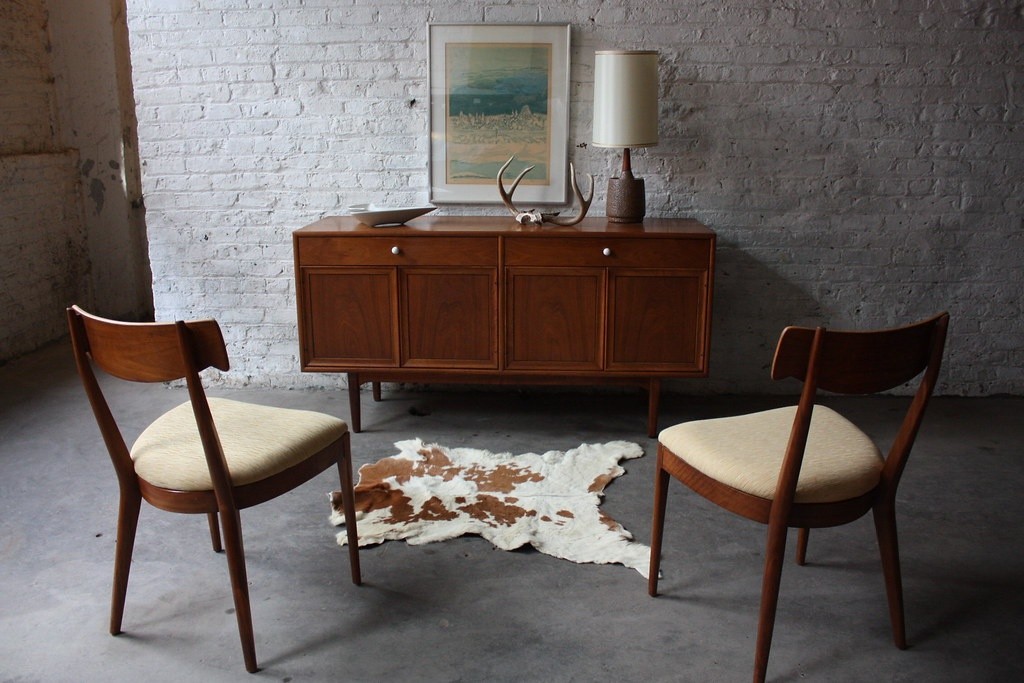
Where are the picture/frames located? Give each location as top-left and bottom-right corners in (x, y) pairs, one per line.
(427, 23), (571, 205)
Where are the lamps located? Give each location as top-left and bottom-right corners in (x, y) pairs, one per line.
(594, 47), (661, 223)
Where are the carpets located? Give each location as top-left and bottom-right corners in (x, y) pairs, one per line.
(328, 436), (663, 580)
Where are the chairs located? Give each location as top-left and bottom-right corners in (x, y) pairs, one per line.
(66, 304), (362, 674)
(647, 311), (951, 683)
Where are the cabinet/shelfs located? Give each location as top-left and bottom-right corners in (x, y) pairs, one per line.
(501, 234), (712, 376)
(298, 234), (499, 369)
